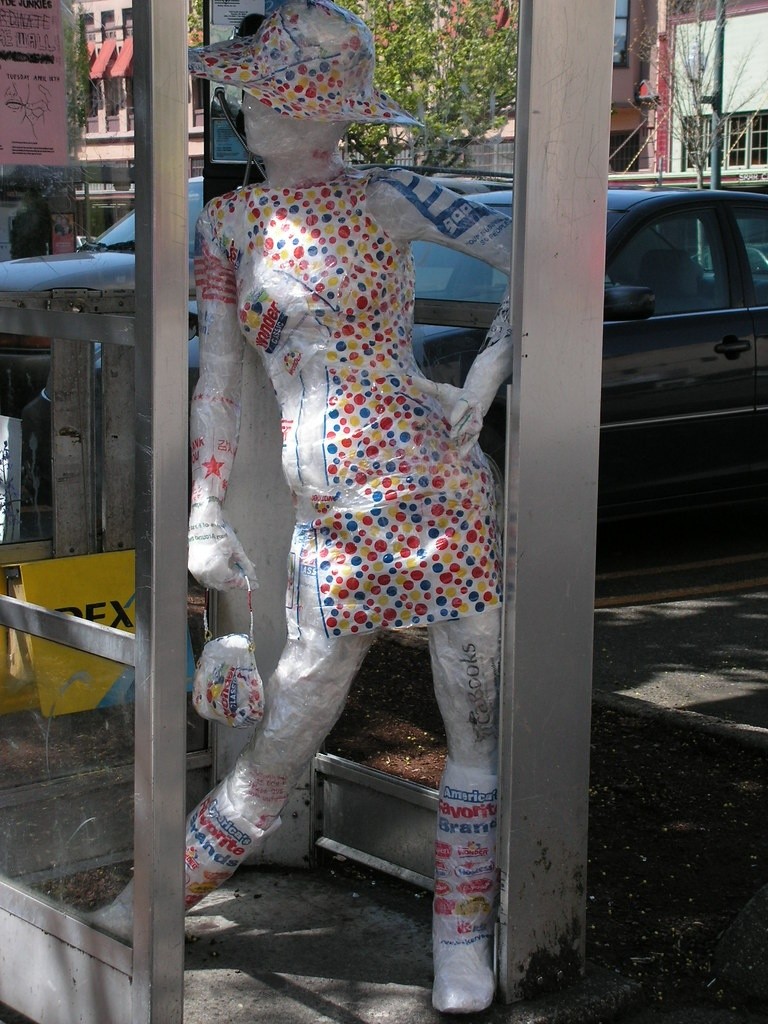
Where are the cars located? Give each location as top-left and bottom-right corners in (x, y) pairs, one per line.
(0, 173), (768, 551)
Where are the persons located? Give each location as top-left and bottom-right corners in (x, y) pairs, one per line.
(85, 3), (517, 1011)
